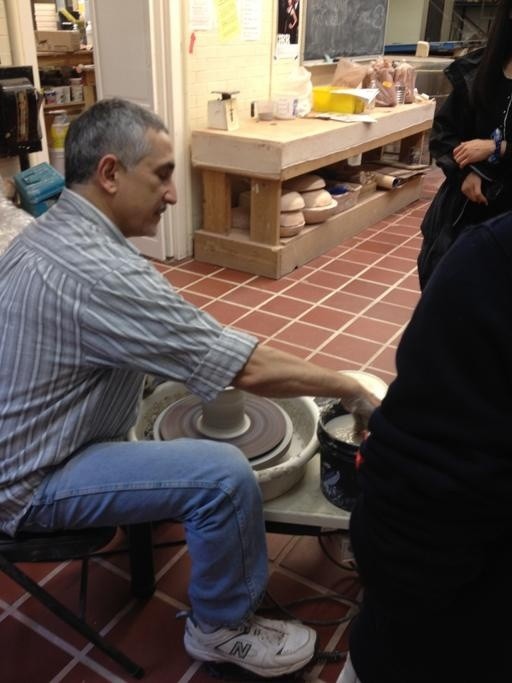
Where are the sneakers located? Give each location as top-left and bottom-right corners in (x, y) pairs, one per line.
(183, 612), (320, 678)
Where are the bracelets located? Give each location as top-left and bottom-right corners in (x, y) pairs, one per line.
(489, 126), (506, 165)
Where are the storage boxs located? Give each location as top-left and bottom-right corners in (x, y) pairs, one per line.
(36, 31), (81, 52)
(313, 86), (365, 115)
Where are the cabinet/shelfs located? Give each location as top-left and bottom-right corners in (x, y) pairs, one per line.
(37, 50), (97, 152)
(191, 101), (436, 279)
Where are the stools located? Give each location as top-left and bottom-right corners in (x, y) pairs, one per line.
(0, 524), (156, 680)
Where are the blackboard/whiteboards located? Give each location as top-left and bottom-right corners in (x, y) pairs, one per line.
(297, 0), (390, 67)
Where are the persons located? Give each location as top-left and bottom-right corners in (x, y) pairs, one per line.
(347, 211), (511, 681)
(417, 1), (512, 290)
(0, 100), (385, 679)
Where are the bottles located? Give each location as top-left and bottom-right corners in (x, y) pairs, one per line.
(86, 22), (93, 46)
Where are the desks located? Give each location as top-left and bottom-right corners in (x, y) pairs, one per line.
(124, 452), (353, 607)
